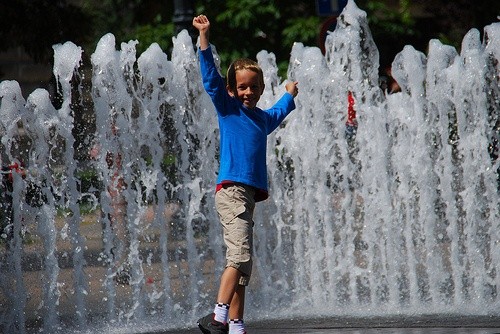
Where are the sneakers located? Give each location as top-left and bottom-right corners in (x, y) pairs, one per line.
(198, 313), (229, 334)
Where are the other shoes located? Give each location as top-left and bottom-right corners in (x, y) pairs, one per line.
(113, 266), (131, 285)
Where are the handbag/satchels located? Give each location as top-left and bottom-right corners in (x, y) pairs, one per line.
(22, 178), (57, 207)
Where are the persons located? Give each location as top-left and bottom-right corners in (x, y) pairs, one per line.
(193, 14), (299, 334)
(346, 66), (403, 163)
(0, 152), (55, 250)
(90, 113), (152, 286)
